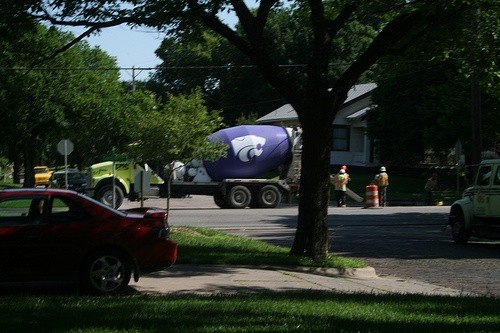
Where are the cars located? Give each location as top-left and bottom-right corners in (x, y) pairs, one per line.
(49, 168), (88, 195)
(0, 187), (178, 293)
(34, 166), (54, 188)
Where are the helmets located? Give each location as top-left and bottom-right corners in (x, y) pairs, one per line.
(380, 166), (386, 171)
(341, 165), (347, 170)
(375, 175), (379, 179)
(339, 169), (345, 173)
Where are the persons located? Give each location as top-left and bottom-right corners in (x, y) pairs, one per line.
(334, 165), (351, 207)
(376, 166), (389, 206)
(424, 172), (438, 205)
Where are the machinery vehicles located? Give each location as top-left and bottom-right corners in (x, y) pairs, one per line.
(86, 125), (303, 208)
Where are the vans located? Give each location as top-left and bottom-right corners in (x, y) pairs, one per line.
(447, 158), (500, 249)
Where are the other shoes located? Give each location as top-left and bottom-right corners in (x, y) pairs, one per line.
(380, 204), (386, 207)
(337, 204), (346, 207)
